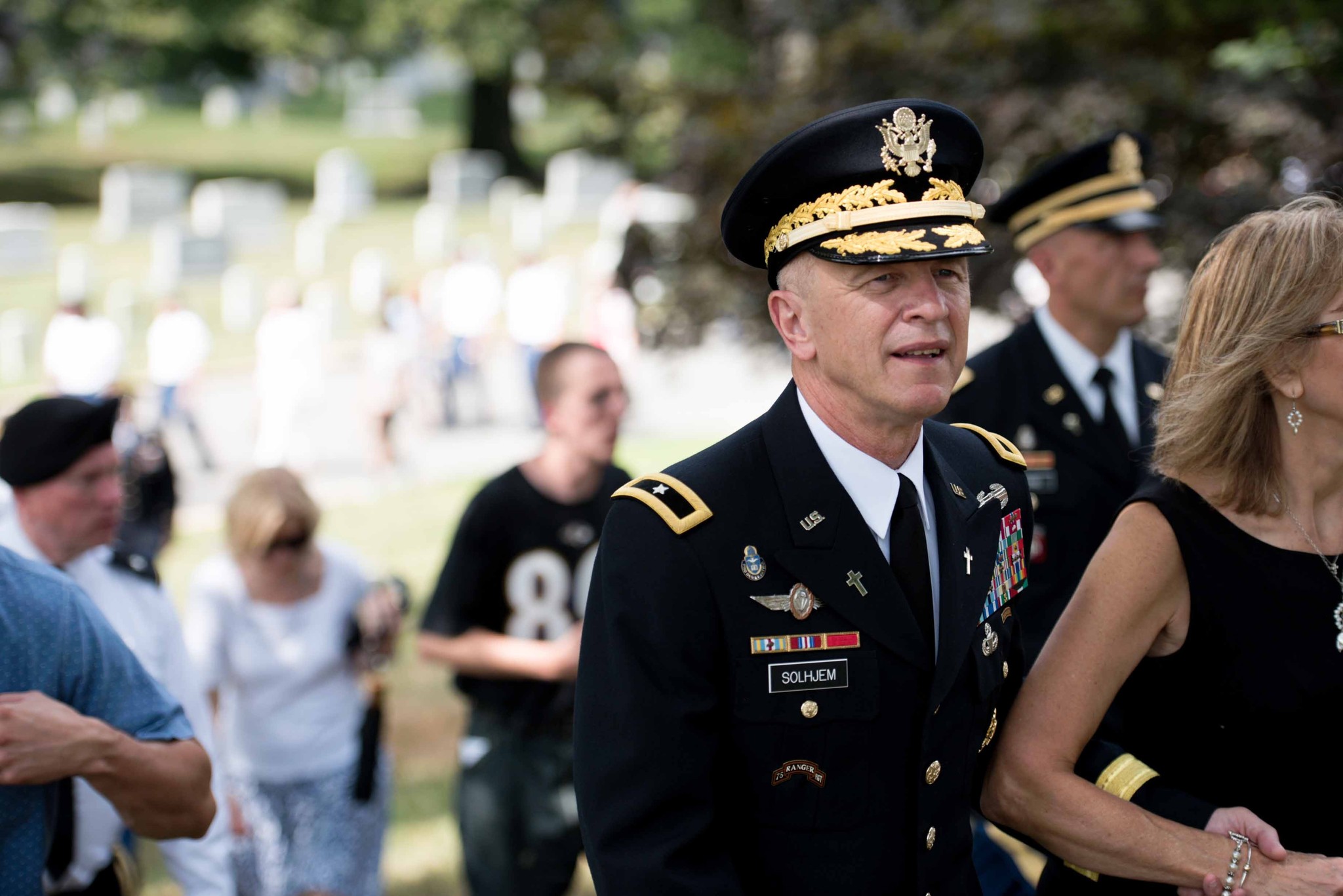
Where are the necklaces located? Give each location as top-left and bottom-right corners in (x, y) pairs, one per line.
(1273, 493), (1343, 653)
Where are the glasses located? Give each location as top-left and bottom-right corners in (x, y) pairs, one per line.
(272, 531), (314, 552)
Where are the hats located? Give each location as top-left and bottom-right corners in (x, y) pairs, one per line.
(0, 391), (125, 486)
(980, 130), (1170, 252)
(720, 95), (996, 262)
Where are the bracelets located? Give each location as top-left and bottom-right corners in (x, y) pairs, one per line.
(1221, 832), (1251, 896)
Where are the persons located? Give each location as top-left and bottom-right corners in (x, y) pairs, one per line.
(574, 99), (1287, 896)
(929, 131), (1177, 896)
(0, 283), (565, 896)
(982, 194), (1343, 896)
(416, 346), (632, 896)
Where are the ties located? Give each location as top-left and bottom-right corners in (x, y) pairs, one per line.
(885, 472), (937, 666)
(46, 776), (82, 881)
(1090, 364), (1130, 447)
